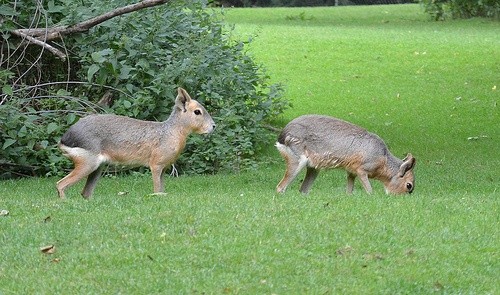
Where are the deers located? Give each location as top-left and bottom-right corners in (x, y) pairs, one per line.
(54, 86), (218, 202)
(272, 113), (417, 199)
